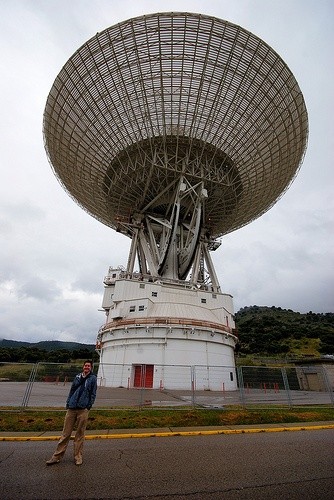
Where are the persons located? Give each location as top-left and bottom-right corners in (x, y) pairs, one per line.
(45, 360), (99, 465)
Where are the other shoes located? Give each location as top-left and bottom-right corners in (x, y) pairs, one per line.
(74, 459), (83, 464)
(46, 458), (60, 464)
